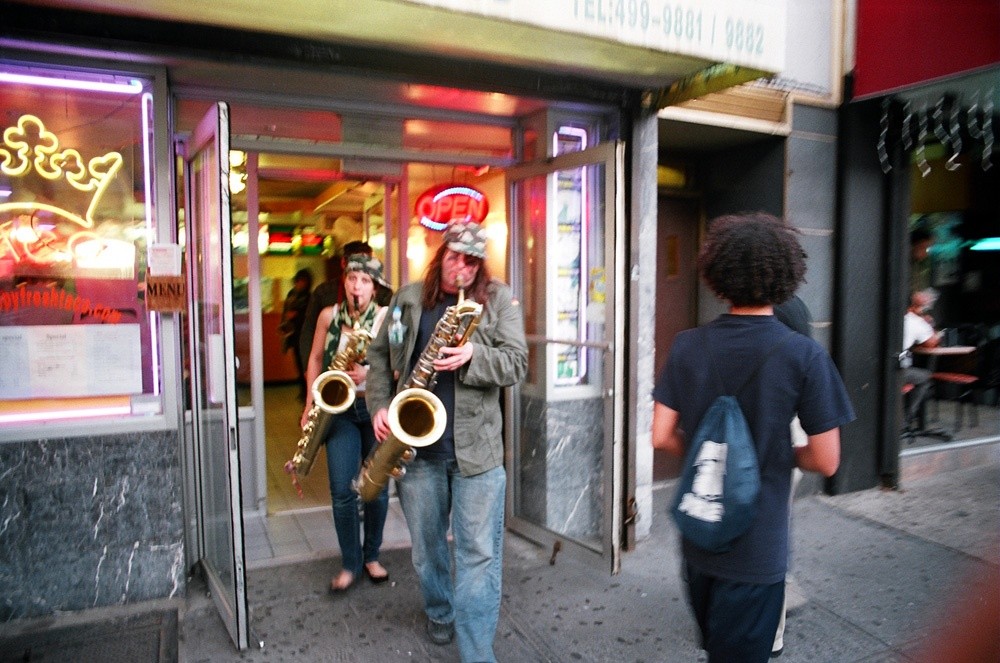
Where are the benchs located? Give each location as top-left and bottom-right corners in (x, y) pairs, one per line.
(920, 370), (978, 431)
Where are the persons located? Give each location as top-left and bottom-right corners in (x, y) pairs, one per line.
(366, 222), (528, 662)
(651, 211), (858, 663)
(280, 241), (400, 594)
(896, 229), (946, 438)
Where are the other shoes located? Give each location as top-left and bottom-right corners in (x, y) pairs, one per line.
(366, 560), (388, 584)
(426, 619), (454, 644)
(332, 569), (360, 593)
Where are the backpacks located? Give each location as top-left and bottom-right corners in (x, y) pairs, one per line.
(669, 329), (799, 550)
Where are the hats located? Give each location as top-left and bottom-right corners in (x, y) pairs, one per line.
(346, 253), (392, 289)
(443, 221), (487, 260)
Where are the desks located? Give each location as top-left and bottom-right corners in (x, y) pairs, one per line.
(910, 344), (977, 443)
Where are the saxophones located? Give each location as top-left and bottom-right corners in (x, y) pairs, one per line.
(350, 275), (484, 501)
(286, 292), (370, 476)
